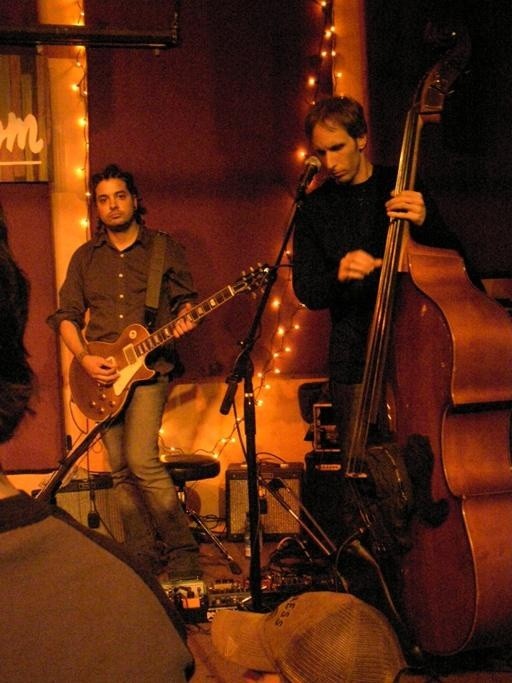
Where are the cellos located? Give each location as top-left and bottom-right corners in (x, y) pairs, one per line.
(341, 44), (512, 657)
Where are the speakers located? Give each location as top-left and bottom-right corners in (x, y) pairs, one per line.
(305, 449), (357, 543)
(225, 462), (303, 544)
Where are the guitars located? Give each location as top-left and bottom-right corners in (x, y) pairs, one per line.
(68, 264), (276, 422)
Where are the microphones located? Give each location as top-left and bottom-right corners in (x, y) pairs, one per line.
(85, 489), (103, 528)
(294, 156), (322, 200)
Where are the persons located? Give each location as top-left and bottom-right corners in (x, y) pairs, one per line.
(289, 95), (487, 448)
(44, 164), (202, 582)
(0, 206), (196, 683)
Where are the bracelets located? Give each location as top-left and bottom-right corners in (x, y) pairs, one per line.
(73, 351), (89, 362)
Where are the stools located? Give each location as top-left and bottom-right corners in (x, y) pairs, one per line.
(159, 453), (242, 574)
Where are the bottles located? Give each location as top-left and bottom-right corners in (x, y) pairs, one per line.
(244, 511), (252, 558)
